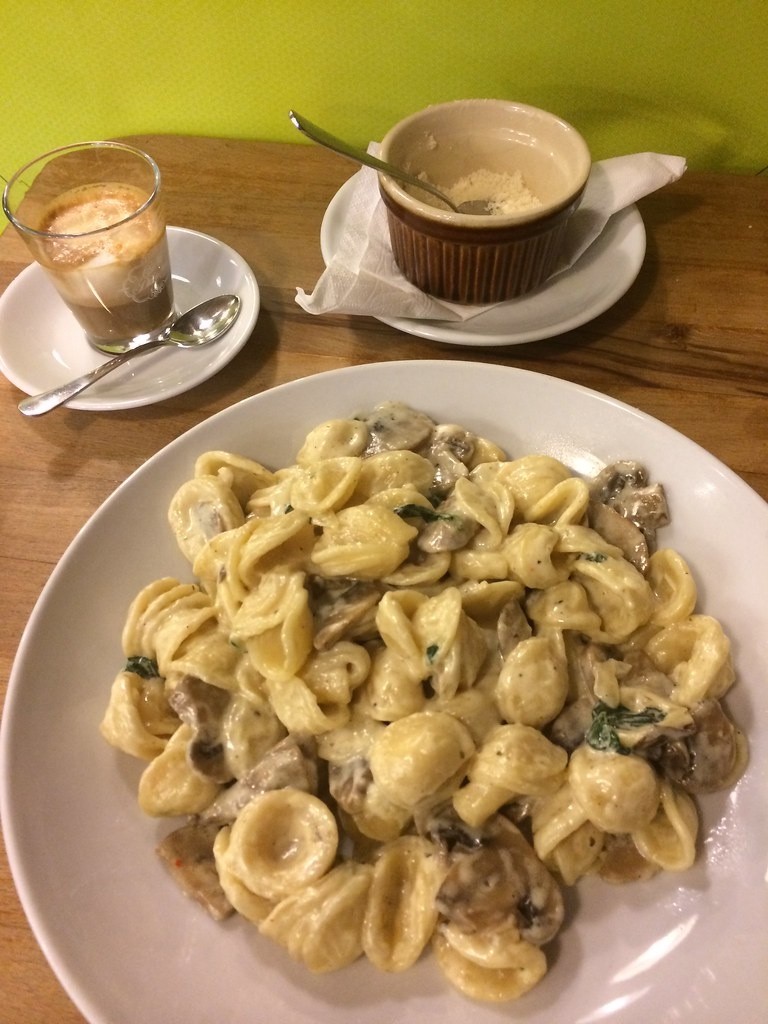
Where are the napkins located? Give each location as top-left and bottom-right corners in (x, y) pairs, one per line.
(294, 138), (689, 322)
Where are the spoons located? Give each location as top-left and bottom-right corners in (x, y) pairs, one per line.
(288, 109), (500, 216)
(18, 295), (242, 416)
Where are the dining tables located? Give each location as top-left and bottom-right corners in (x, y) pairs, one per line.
(2, 134), (768, 1024)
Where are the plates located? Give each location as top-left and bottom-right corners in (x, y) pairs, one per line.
(1, 359), (767, 1022)
(320, 165), (647, 347)
(1, 225), (260, 410)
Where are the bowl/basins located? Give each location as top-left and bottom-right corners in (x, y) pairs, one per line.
(377, 98), (591, 305)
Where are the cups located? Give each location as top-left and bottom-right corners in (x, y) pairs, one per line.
(1, 140), (180, 357)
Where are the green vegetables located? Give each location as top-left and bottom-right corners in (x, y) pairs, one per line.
(124, 502), (667, 756)
